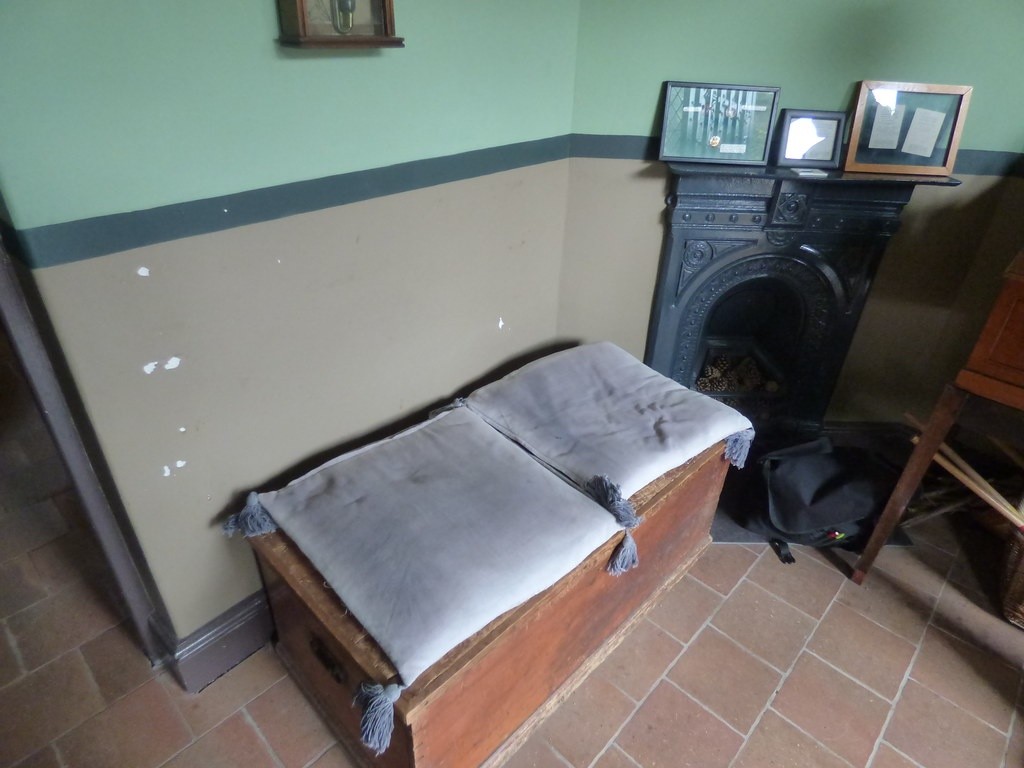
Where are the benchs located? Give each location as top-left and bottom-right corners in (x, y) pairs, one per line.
(223, 339), (755, 768)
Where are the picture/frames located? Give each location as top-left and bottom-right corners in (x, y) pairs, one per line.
(845, 79), (974, 176)
(776, 109), (846, 169)
(659, 81), (781, 167)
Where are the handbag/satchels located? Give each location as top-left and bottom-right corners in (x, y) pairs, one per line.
(752, 437), (909, 563)
